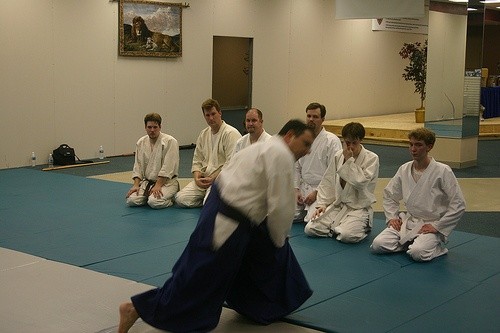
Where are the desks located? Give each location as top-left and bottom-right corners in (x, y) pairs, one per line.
(479, 85), (500, 119)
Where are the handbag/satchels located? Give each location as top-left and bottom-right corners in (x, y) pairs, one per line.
(52, 144), (93, 166)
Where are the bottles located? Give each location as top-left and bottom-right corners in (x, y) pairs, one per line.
(99, 146), (104, 159)
(48, 153), (53, 168)
(32, 152), (36, 167)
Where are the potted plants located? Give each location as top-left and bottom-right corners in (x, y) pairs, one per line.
(398, 39), (427, 124)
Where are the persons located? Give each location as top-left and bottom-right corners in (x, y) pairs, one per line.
(369, 128), (466, 262)
(174, 99), (242, 209)
(231, 109), (272, 159)
(118, 120), (313, 333)
(290, 102), (379, 244)
(125, 112), (181, 208)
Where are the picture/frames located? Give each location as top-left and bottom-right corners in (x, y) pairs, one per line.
(117, 0), (184, 57)
